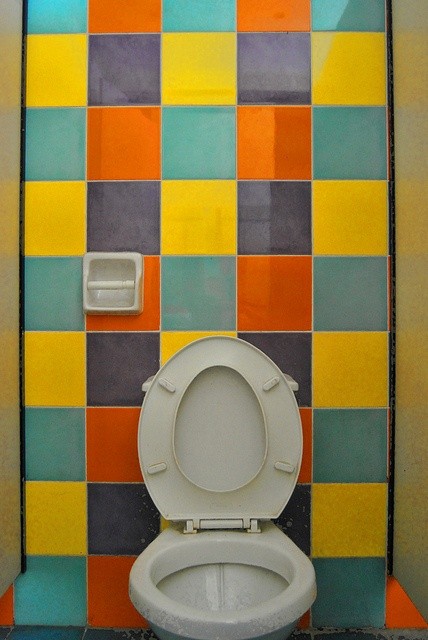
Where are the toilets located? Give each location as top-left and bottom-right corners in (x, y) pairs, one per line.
(127, 335), (319, 640)
